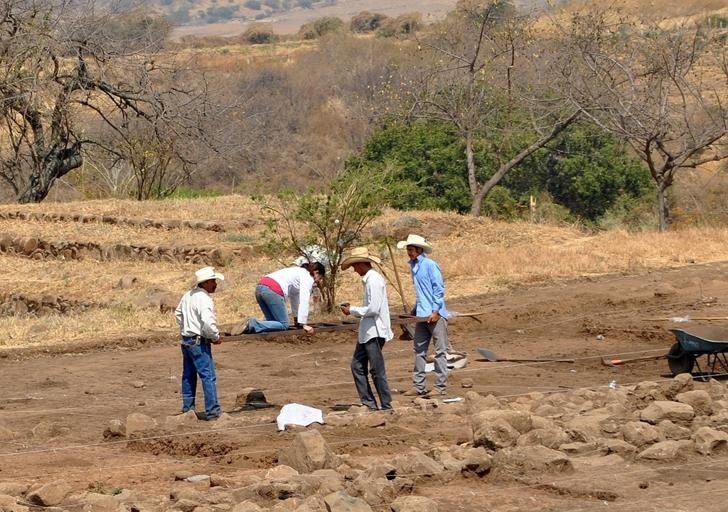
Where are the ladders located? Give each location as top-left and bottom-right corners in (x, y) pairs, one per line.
(222, 314), (430, 342)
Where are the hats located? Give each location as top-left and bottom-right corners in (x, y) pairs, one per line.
(191, 267), (225, 289)
(397, 233), (432, 255)
(341, 247), (381, 270)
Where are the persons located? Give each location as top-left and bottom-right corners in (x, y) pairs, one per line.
(229, 261), (327, 336)
(173, 265), (226, 423)
(338, 245), (397, 411)
(395, 234), (450, 398)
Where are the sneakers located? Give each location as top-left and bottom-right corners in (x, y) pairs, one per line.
(404, 387), (447, 396)
(230, 315), (255, 336)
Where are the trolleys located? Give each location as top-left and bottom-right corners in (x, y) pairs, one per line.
(663, 327), (728, 381)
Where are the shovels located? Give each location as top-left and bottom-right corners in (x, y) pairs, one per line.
(385, 236), (415, 341)
(477, 348), (575, 363)
